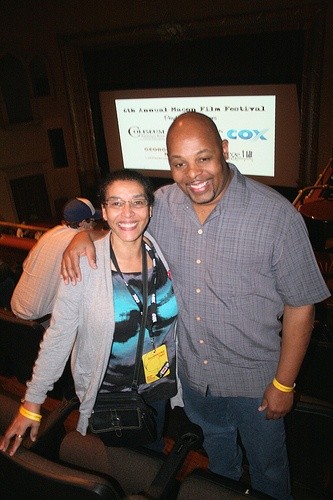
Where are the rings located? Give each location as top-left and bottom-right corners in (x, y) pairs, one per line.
(16, 434), (23, 438)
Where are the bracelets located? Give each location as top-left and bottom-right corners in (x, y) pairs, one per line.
(272, 376), (295, 393)
(19, 404), (42, 422)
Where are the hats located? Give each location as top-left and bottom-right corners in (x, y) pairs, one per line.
(64, 197), (103, 221)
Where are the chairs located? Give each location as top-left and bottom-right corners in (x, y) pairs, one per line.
(0, 233), (333, 500)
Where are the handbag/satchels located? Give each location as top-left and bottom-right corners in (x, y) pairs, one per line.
(89, 391), (164, 453)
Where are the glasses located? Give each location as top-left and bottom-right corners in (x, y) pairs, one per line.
(103, 200), (154, 209)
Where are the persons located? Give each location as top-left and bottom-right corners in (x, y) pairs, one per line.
(61, 111), (331, 500)
(11, 197), (101, 400)
(0, 169), (184, 455)
